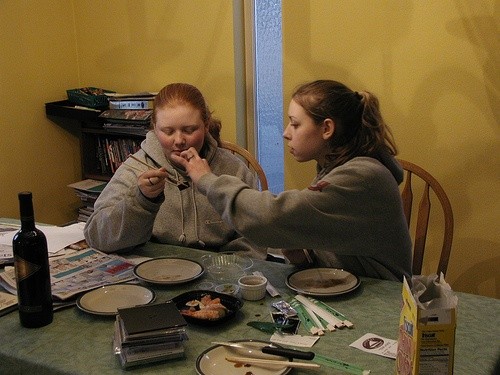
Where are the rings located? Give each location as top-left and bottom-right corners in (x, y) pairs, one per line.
(148, 178), (154, 185)
(188, 154), (194, 160)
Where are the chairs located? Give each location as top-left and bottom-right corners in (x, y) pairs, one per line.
(217, 139), (454, 283)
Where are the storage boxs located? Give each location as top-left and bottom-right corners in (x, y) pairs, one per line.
(46, 86), (116, 123)
(396, 272), (458, 375)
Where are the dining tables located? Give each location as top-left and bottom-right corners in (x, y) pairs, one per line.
(0, 218), (500, 375)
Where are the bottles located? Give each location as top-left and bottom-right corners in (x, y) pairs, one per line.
(12, 191), (54, 329)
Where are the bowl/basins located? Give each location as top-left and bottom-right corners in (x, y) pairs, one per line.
(215, 284), (239, 297)
(200, 253), (255, 281)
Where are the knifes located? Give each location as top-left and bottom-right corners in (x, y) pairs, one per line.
(210, 342), (315, 360)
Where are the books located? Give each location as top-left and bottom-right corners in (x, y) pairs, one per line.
(94, 136), (142, 175)
(68, 178), (108, 222)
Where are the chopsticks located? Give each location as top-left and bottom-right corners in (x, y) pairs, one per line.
(311, 322), (353, 336)
(225, 357), (322, 368)
(123, 154), (188, 188)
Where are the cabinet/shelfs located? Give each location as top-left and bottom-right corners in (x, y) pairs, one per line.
(77, 125), (156, 208)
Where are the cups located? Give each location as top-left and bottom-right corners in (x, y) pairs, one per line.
(238, 275), (268, 300)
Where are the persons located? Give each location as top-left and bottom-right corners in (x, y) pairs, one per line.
(170, 79), (415, 284)
(83, 83), (268, 260)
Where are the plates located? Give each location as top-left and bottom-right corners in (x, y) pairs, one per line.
(75, 283), (156, 316)
(132, 256), (206, 284)
(166, 289), (245, 325)
(193, 339), (293, 375)
(285, 266), (362, 296)
